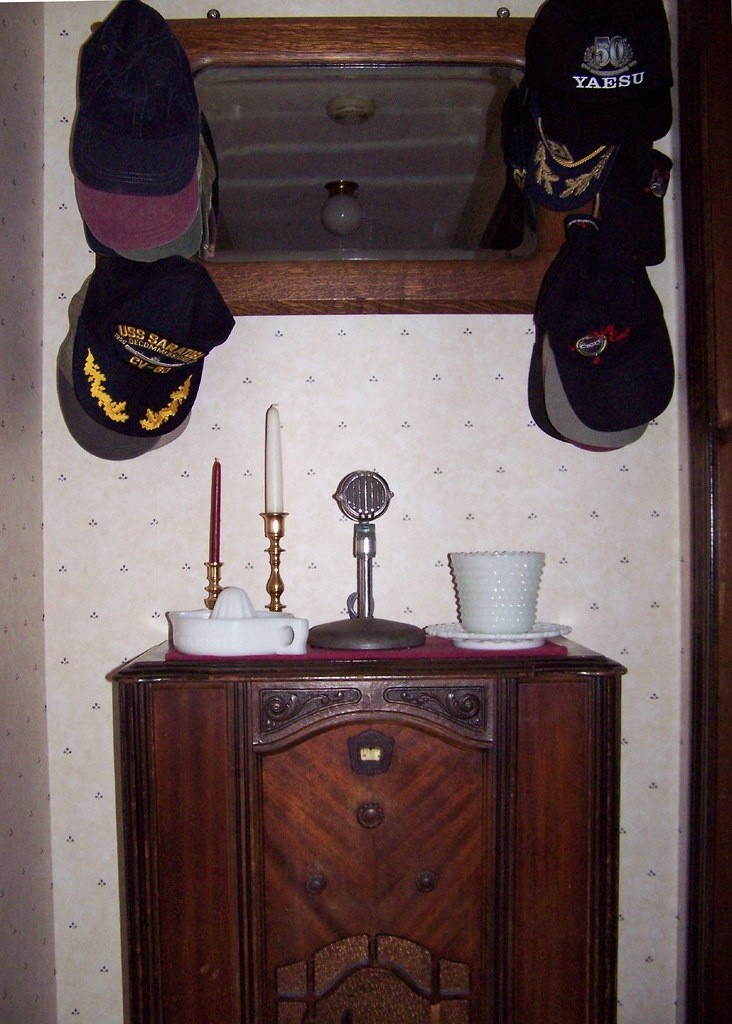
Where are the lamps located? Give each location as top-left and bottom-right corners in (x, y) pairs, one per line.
(322, 181), (363, 236)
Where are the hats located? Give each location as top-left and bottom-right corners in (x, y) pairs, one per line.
(69, 0), (202, 194)
(55, 253), (237, 461)
(476, 0), (673, 264)
(71, 114), (220, 261)
(527, 211), (678, 451)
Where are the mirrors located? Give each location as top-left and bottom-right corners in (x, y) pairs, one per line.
(163, 17), (602, 316)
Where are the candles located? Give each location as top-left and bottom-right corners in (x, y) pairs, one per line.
(209, 458), (221, 563)
(266, 404), (284, 513)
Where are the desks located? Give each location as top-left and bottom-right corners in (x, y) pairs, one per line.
(104, 636), (627, 1024)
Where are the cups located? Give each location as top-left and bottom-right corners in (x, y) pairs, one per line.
(448, 549), (547, 635)
(165, 607), (310, 658)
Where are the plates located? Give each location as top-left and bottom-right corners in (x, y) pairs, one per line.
(425, 620), (572, 650)
